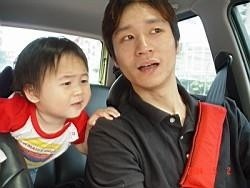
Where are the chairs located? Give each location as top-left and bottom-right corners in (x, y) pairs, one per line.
(0, 83), (111, 188)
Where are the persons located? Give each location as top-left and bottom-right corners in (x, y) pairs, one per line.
(84, 0), (250, 188)
(0, 37), (121, 186)
